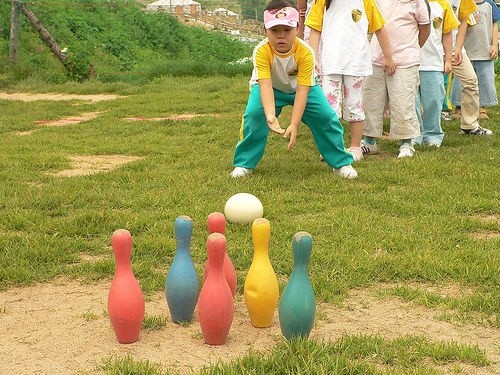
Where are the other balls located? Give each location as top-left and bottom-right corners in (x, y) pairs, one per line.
(223, 193), (263, 226)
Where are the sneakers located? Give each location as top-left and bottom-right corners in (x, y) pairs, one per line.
(346, 149), (364, 162)
(320, 154), (326, 162)
(411, 139), (423, 147)
(360, 140), (377, 155)
(478, 107), (489, 119)
(427, 142), (441, 148)
(397, 142), (415, 159)
(458, 125), (492, 136)
(230, 167), (254, 179)
(441, 112), (455, 121)
(332, 164), (359, 179)
(452, 109), (461, 118)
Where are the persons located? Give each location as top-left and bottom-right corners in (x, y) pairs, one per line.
(229, 1), (358, 180)
(297, 0), (500, 163)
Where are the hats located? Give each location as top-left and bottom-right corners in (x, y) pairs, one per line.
(263, 6), (299, 29)
